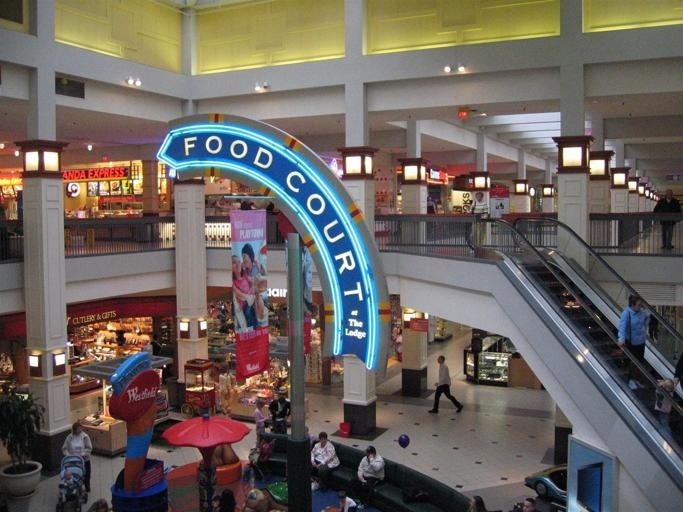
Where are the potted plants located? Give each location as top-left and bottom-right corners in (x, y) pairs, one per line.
(0, 372), (46, 498)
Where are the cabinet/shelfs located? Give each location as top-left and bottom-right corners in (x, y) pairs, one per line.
(462, 333), (545, 393)
(0, 304), (324, 458)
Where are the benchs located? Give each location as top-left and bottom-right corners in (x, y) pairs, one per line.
(253, 431), (472, 512)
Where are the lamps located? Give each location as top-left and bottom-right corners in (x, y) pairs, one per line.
(336, 145), (381, 181)
(253, 81), (269, 91)
(468, 170), (491, 190)
(13, 138), (70, 178)
(444, 62), (466, 74)
(396, 157), (430, 186)
(551, 135), (661, 201)
(540, 183), (555, 198)
(512, 178), (530, 195)
(126, 77), (143, 86)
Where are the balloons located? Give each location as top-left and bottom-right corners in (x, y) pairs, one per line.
(399, 435), (410, 449)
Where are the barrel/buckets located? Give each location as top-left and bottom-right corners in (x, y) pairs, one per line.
(340, 422), (351, 437)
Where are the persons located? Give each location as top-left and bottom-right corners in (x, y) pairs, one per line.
(513, 498), (543, 512)
(652, 188), (683, 250)
(70, 184), (80, 197)
(310, 431), (341, 491)
(469, 495), (489, 512)
(232, 242), (268, 334)
(210, 301), (235, 339)
(303, 261), (313, 317)
(60, 469), (79, 503)
(618, 294), (683, 437)
(248, 396), (291, 481)
(350, 445), (386, 509)
(470, 192), (489, 248)
(213, 489), (242, 512)
(337, 490), (358, 512)
(427, 196), (437, 234)
(428, 356), (463, 413)
(61, 422), (93, 492)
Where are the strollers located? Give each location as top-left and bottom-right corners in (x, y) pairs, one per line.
(54, 454), (91, 511)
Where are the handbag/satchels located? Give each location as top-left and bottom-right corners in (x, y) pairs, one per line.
(621, 340), (633, 361)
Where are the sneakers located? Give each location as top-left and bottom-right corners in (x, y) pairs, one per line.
(456, 405), (464, 413)
(428, 409), (439, 414)
(629, 379), (646, 391)
